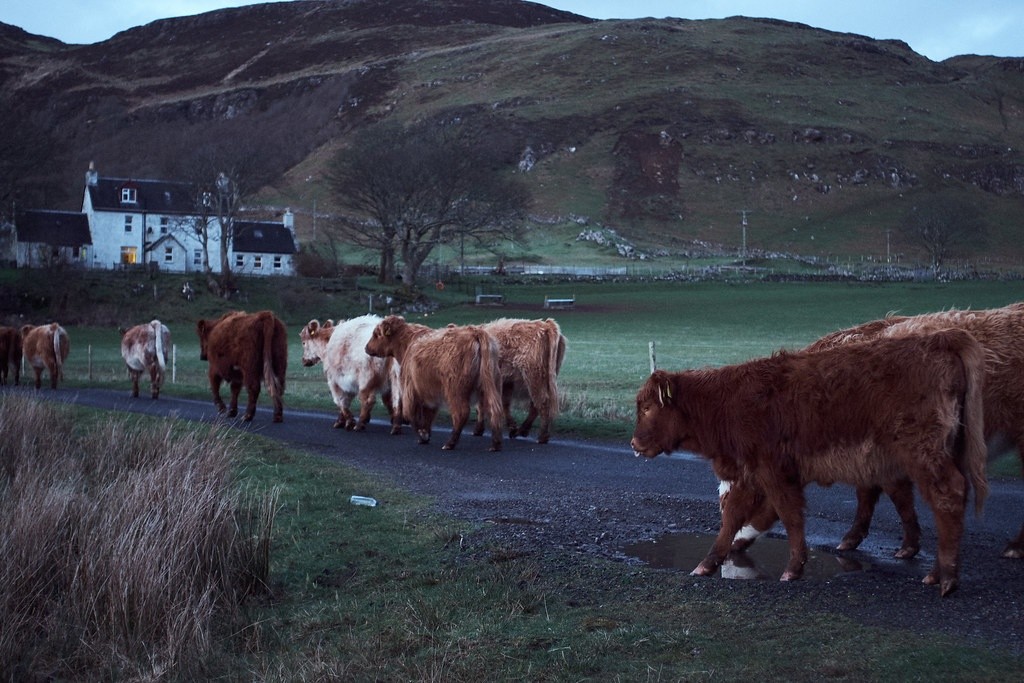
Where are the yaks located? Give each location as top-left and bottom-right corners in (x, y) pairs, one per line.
(630, 301), (1024, 597)
(0, 311), (567, 453)
